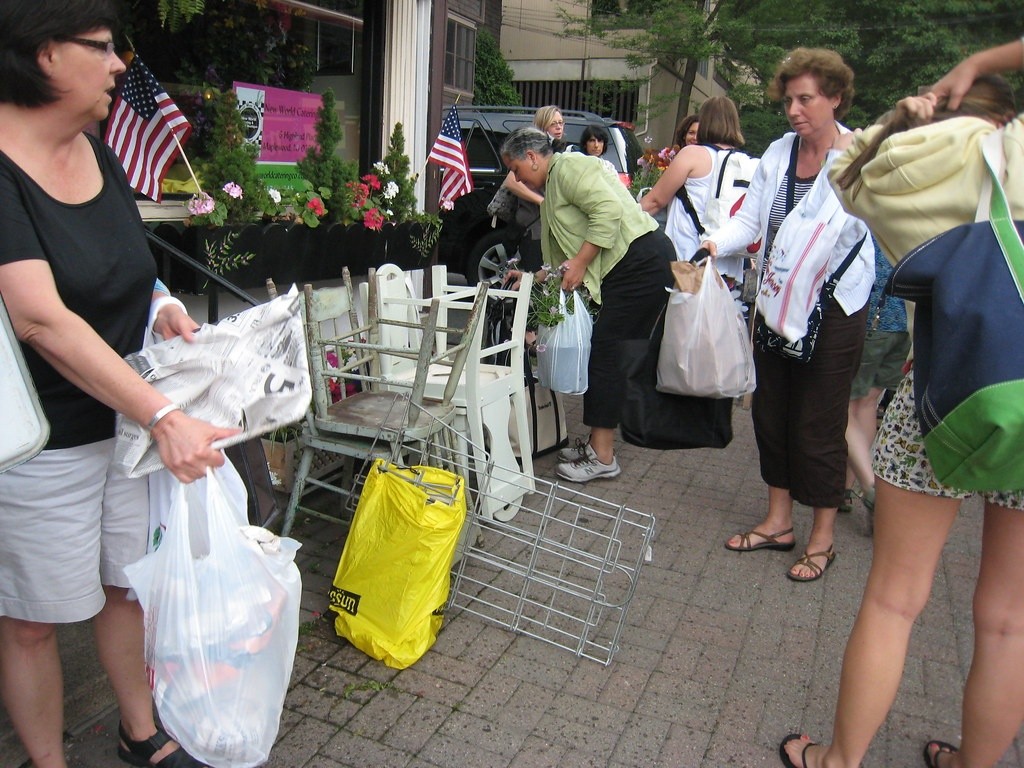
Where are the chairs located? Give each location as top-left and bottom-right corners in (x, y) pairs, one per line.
(284, 263), (532, 568)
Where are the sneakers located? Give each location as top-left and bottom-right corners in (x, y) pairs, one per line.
(556, 434), (597, 462)
(556, 445), (621, 482)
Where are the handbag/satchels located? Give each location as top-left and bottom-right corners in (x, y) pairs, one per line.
(656, 254), (758, 399)
(0, 295), (49, 476)
(888, 127), (1024, 492)
(534, 284), (593, 395)
(757, 187), (848, 342)
(122, 468), (302, 768)
(486, 180), (516, 224)
(620, 251), (734, 450)
(328, 459), (467, 670)
(754, 294), (824, 378)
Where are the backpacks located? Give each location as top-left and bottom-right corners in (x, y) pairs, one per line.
(675, 142), (762, 256)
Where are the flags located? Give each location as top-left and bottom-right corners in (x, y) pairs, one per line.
(102, 50), (193, 204)
(428, 105), (473, 211)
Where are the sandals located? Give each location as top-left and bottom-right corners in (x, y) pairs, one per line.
(780, 735), (820, 768)
(725, 528), (795, 551)
(118, 724), (202, 767)
(786, 544), (836, 582)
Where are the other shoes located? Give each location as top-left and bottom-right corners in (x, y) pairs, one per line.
(837, 489), (853, 513)
(862, 491), (875, 509)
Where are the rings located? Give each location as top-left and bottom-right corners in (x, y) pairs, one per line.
(571, 286), (575, 290)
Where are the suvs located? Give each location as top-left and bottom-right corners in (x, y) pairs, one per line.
(441, 104), (649, 314)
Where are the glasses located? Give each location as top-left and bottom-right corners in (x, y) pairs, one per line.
(550, 121), (564, 129)
(64, 35), (114, 57)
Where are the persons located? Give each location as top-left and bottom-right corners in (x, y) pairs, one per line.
(483, 102), (678, 483)
(778, 35), (1024, 768)
(701, 50), (878, 583)
(636, 96), (759, 289)
(0, 0), (239, 768)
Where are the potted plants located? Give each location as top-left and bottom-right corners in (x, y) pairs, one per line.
(261, 401), (344, 492)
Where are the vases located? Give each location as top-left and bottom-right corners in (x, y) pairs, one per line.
(187, 223), (437, 294)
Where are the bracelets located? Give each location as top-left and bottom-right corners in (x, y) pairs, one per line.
(146, 403), (182, 432)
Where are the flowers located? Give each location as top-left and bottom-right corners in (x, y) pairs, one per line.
(630, 137), (681, 203)
(182, 161), (445, 257)
(507, 258), (568, 352)
(325, 337), (366, 403)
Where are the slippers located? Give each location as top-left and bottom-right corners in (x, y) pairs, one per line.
(924, 739), (959, 767)
